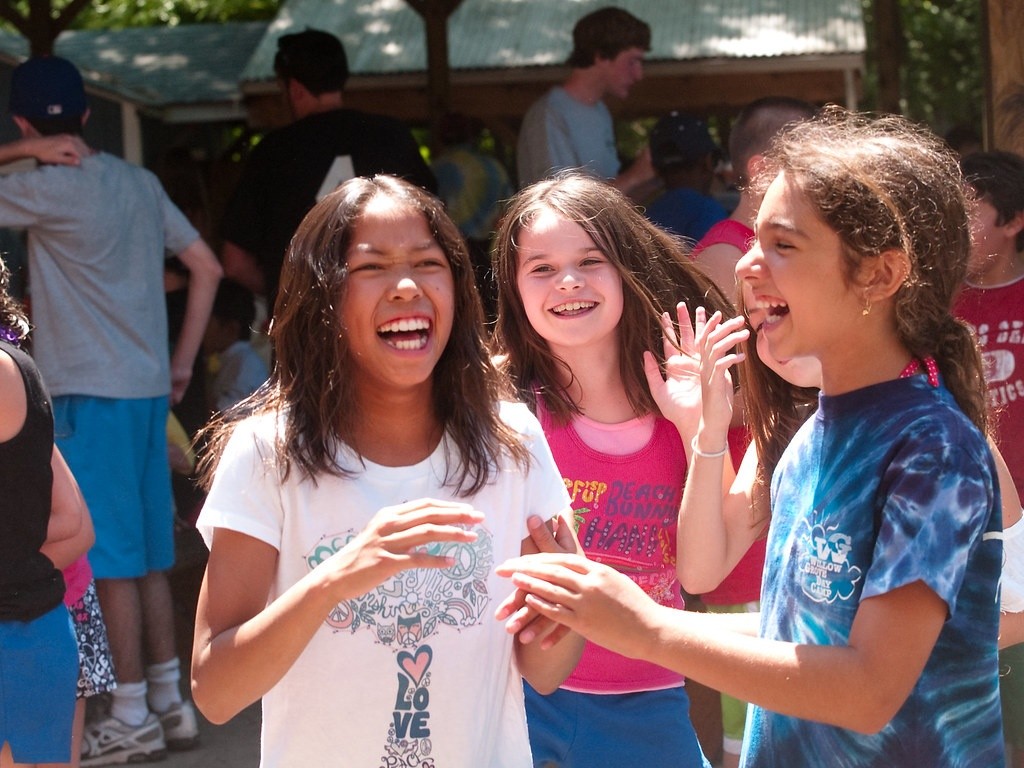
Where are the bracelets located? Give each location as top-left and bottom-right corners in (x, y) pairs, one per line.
(691, 436), (728, 458)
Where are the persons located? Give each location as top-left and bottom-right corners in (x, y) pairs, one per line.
(191, 176), (585, 768)
(492, 177), (736, 768)
(497, 128), (1008, 768)
(645, 96), (1024, 768)
(0, 29), (437, 768)
(518, 8), (651, 196)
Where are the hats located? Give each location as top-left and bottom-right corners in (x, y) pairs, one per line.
(11, 54), (87, 116)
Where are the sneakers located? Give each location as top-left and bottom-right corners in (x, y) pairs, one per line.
(150, 700), (201, 751)
(80, 709), (166, 767)
